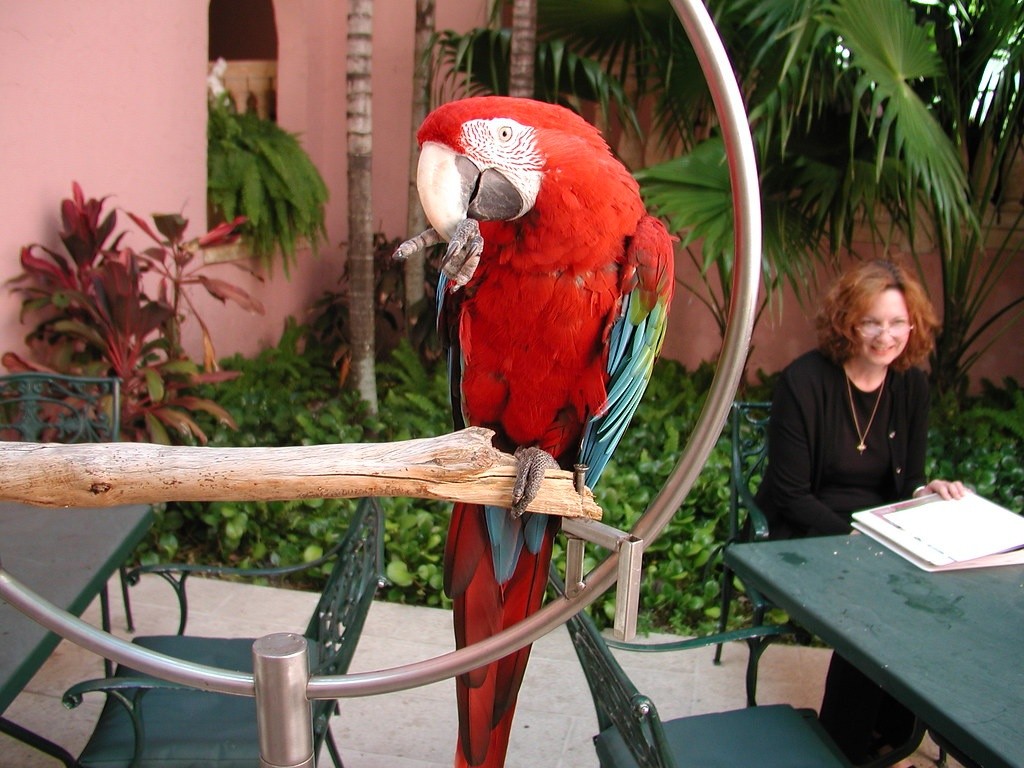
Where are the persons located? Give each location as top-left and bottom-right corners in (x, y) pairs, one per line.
(736, 255), (965, 768)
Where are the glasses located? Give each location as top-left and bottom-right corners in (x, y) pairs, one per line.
(854, 316), (914, 337)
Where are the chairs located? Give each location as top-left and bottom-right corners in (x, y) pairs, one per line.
(713, 399), (950, 764)
(0, 370), (136, 678)
(549, 561), (855, 768)
(62, 495), (384, 768)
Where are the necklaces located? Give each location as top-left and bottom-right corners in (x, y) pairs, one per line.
(843, 365), (886, 455)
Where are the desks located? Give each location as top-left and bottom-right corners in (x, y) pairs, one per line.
(721, 534), (1024, 768)
(0, 498), (156, 768)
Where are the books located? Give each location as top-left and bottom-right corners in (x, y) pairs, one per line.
(850, 489), (1024, 573)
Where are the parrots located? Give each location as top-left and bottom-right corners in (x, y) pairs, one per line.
(414, 94), (681, 768)
